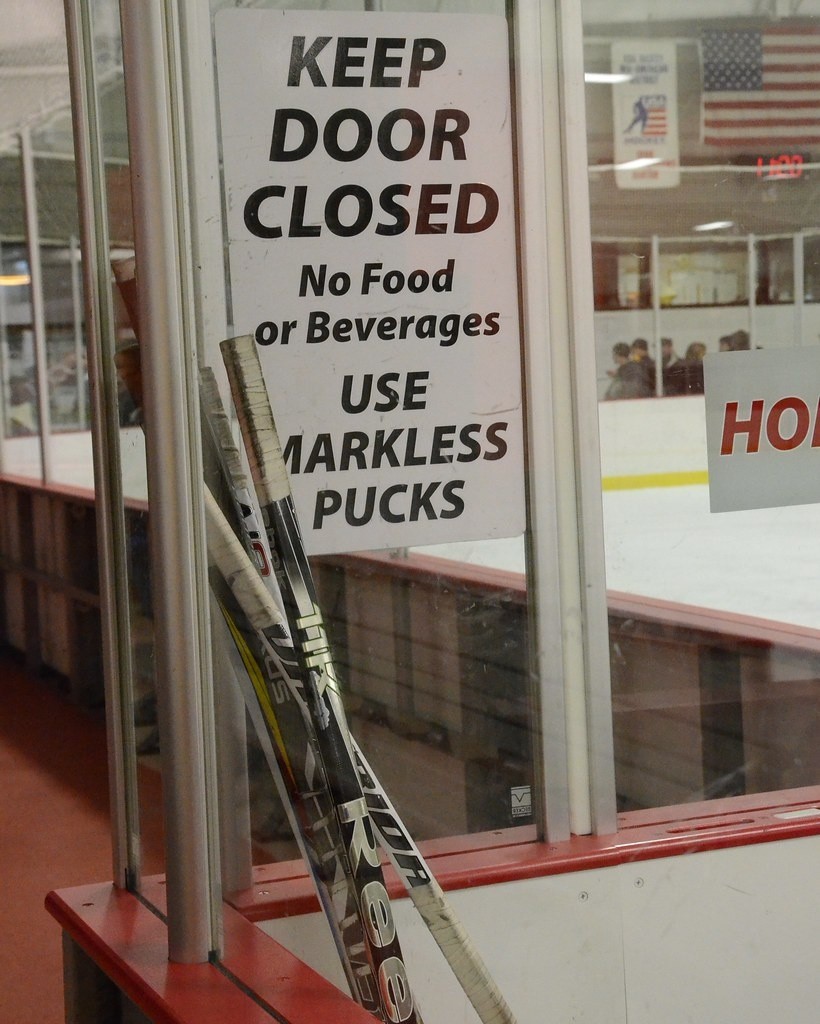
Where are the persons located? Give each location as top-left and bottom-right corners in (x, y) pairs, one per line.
(602, 329), (765, 401)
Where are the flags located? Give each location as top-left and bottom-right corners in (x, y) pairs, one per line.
(695, 27), (820, 149)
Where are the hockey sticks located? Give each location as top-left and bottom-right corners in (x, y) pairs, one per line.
(111, 255), (518, 1024)
